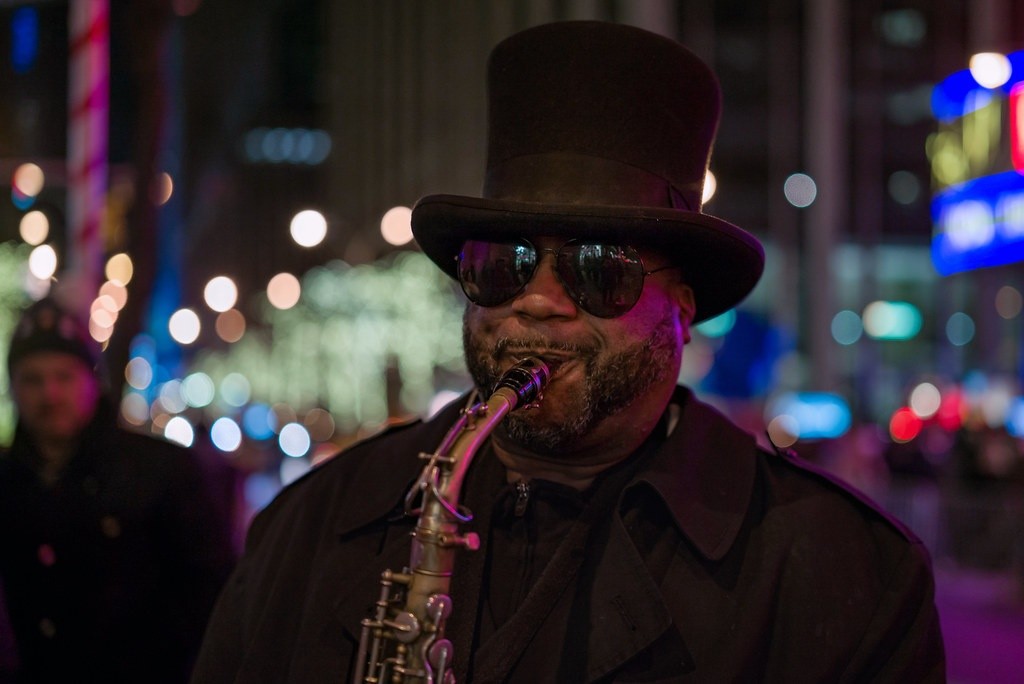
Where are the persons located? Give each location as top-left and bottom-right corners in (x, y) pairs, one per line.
(0, 296), (226, 684)
(194, 21), (945, 684)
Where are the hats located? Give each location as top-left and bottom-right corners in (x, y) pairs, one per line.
(412, 21), (765, 327)
(7, 298), (110, 393)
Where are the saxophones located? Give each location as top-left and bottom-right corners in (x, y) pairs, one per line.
(354, 353), (572, 684)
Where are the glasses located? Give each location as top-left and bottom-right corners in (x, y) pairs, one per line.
(441, 235), (677, 318)
